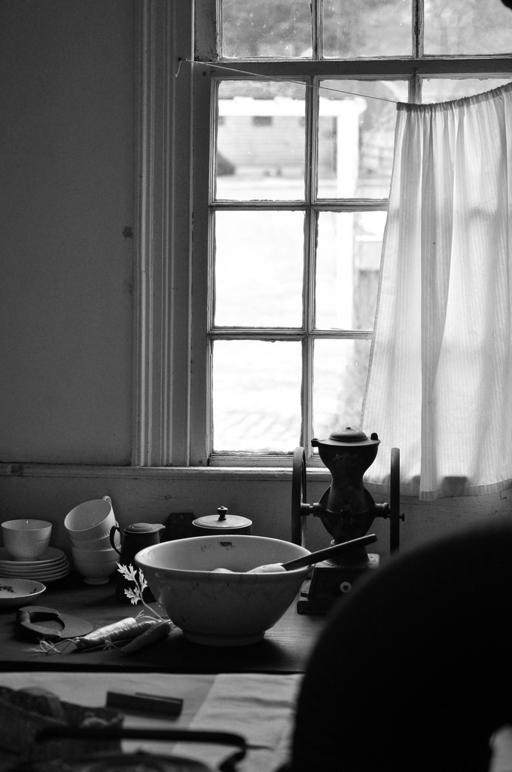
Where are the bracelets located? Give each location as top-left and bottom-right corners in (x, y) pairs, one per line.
(134, 534), (314, 652)
(2, 518), (53, 560)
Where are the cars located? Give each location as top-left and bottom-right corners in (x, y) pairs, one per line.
(191, 506), (252, 538)
(65, 496), (120, 586)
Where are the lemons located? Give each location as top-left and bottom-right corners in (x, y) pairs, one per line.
(109, 522), (166, 603)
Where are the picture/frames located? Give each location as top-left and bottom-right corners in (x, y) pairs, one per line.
(0, 546), (322, 678)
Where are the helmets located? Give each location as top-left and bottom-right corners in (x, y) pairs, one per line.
(0, 578), (46, 608)
(0, 547), (69, 581)
